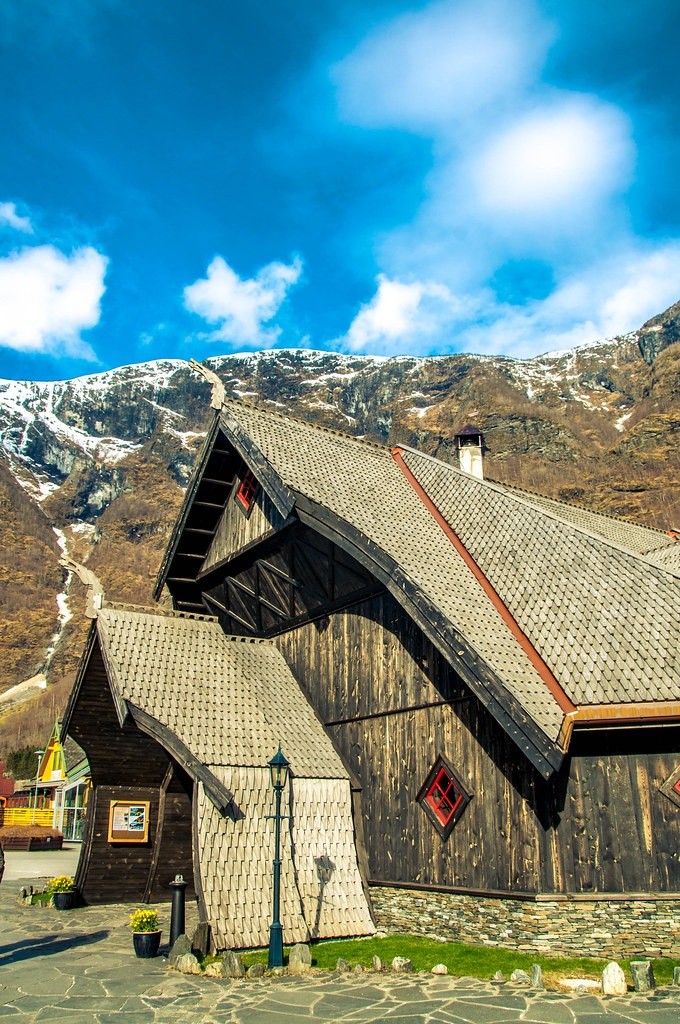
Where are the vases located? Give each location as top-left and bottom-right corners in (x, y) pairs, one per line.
(52, 891), (75, 910)
(130, 928), (163, 959)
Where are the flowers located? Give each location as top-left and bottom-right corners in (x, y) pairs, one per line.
(46, 874), (74, 893)
(129, 906), (164, 934)
